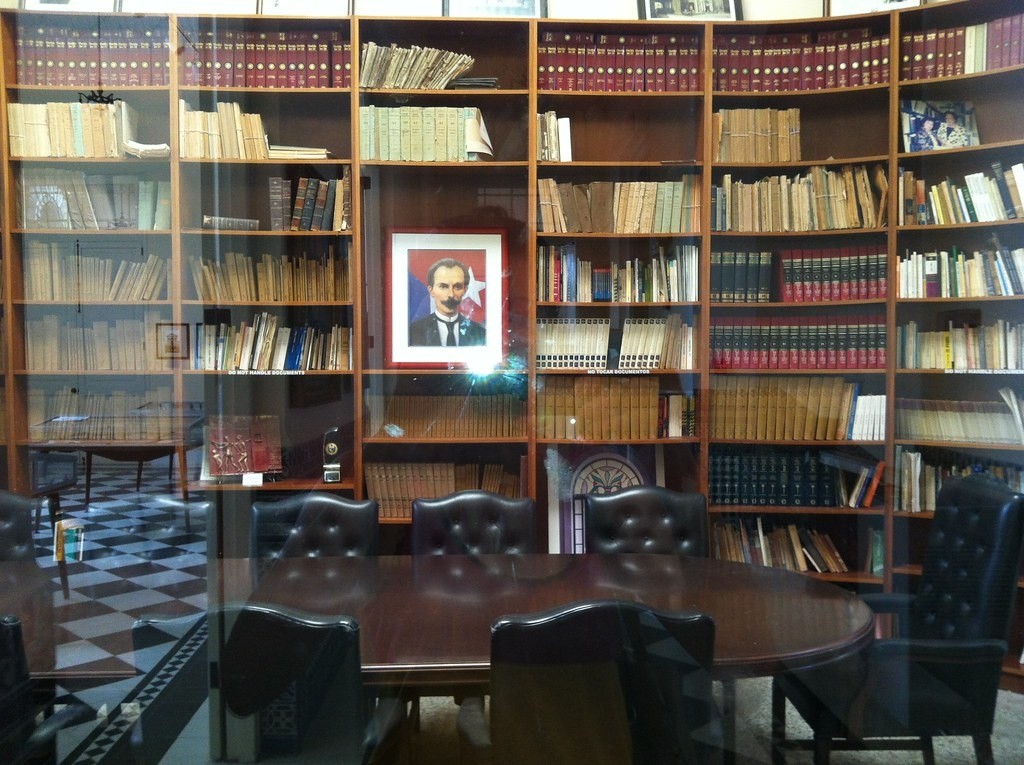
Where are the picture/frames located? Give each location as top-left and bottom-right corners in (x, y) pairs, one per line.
(156, 323), (190, 360)
(384, 225), (509, 373)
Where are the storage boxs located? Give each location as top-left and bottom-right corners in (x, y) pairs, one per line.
(637, 0), (744, 20)
(442, 0), (548, 18)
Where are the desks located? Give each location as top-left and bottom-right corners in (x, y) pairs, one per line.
(1, 553), (877, 765)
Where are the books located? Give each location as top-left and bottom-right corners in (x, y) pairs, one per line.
(0, 19), (1024, 647)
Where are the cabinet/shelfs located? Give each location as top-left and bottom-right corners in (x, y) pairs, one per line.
(0, 7), (1024, 698)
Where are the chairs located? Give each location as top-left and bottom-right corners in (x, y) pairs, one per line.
(410, 489), (537, 558)
(457, 600), (717, 765)
(582, 486), (711, 558)
(772, 475), (1024, 765)
(0, 615), (96, 765)
(0, 491), (58, 765)
(131, 603), (420, 765)
(247, 493), (378, 588)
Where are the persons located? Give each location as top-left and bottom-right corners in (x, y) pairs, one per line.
(908, 116), (939, 151)
(408, 257), (487, 346)
(937, 111), (972, 147)
